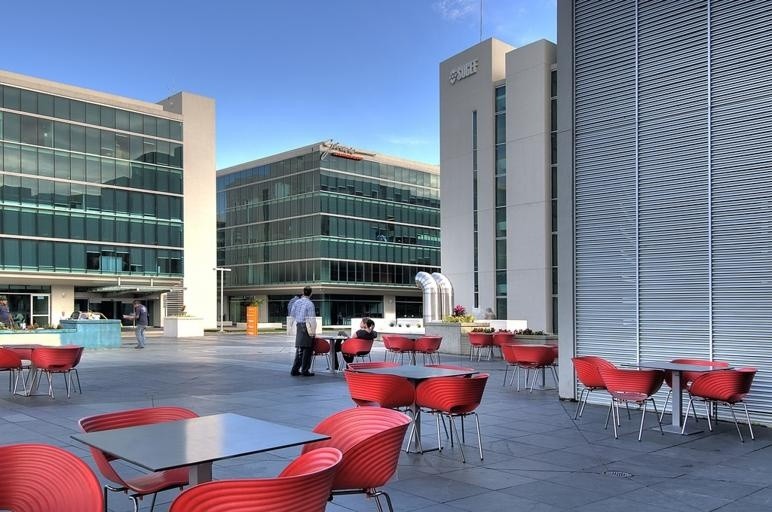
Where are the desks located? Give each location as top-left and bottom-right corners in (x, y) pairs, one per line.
(70, 412), (332, 488)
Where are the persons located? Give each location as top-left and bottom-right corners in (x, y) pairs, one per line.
(289, 286), (317, 376)
(327, 317), (377, 370)
(0, 295), (24, 330)
(123, 300), (148, 349)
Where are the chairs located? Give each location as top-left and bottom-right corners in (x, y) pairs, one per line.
(301, 407), (413, 512)
(0, 344), (84, 400)
(0, 444), (105, 512)
(167, 447), (343, 511)
(78, 406), (202, 512)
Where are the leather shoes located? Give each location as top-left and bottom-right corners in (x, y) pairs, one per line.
(291, 370), (302, 376)
(303, 371), (314, 376)
